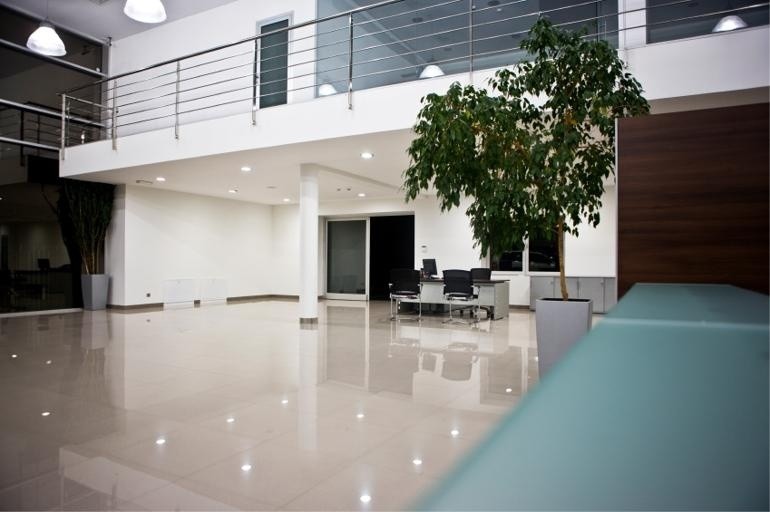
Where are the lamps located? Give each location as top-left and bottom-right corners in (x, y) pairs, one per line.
(416, 1), (446, 80)
(26, 0), (69, 59)
(710, 1), (749, 34)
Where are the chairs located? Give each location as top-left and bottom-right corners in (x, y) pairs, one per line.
(390, 267), (491, 322)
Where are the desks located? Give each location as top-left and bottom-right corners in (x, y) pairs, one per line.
(390, 277), (511, 320)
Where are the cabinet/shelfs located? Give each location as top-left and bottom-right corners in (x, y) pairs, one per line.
(528, 275), (616, 313)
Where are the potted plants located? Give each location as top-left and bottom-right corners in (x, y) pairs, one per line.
(395, 13), (653, 389)
(56, 182), (113, 311)
